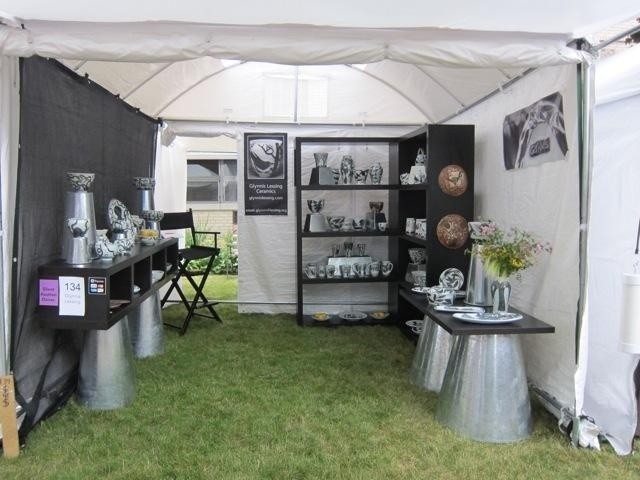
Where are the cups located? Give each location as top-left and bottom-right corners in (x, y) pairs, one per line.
(381, 261), (393, 276)
(339, 265), (351, 277)
(318, 266), (325, 277)
(378, 223), (387, 231)
(352, 263), (366, 277)
(342, 222), (351, 230)
(326, 265), (335, 277)
(368, 262), (380, 277)
(304, 263), (316, 279)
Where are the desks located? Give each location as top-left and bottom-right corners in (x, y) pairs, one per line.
(400, 288), (555, 445)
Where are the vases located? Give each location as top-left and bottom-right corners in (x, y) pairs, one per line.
(491, 278), (512, 314)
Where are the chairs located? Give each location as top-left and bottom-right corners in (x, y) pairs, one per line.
(158, 211), (223, 335)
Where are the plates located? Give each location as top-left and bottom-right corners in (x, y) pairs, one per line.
(411, 326), (423, 333)
(434, 306), (485, 313)
(312, 315), (329, 321)
(412, 287), (429, 294)
(339, 312), (367, 321)
(453, 312), (523, 324)
(370, 312), (389, 320)
(438, 267), (464, 290)
(406, 320), (424, 327)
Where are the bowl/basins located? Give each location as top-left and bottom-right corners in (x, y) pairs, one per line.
(370, 202), (383, 212)
(408, 248), (426, 264)
(68, 219), (91, 238)
(139, 236), (158, 245)
(328, 216), (344, 228)
(134, 177), (155, 189)
(142, 210), (163, 221)
(152, 271), (164, 280)
(67, 173), (95, 191)
(307, 199), (324, 213)
(412, 272), (426, 286)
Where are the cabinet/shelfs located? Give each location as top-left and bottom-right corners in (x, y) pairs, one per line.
(295, 137), (400, 327)
(399, 124), (475, 342)
(35, 238), (179, 410)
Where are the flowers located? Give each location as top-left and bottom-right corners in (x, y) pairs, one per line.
(471, 221), (553, 281)
